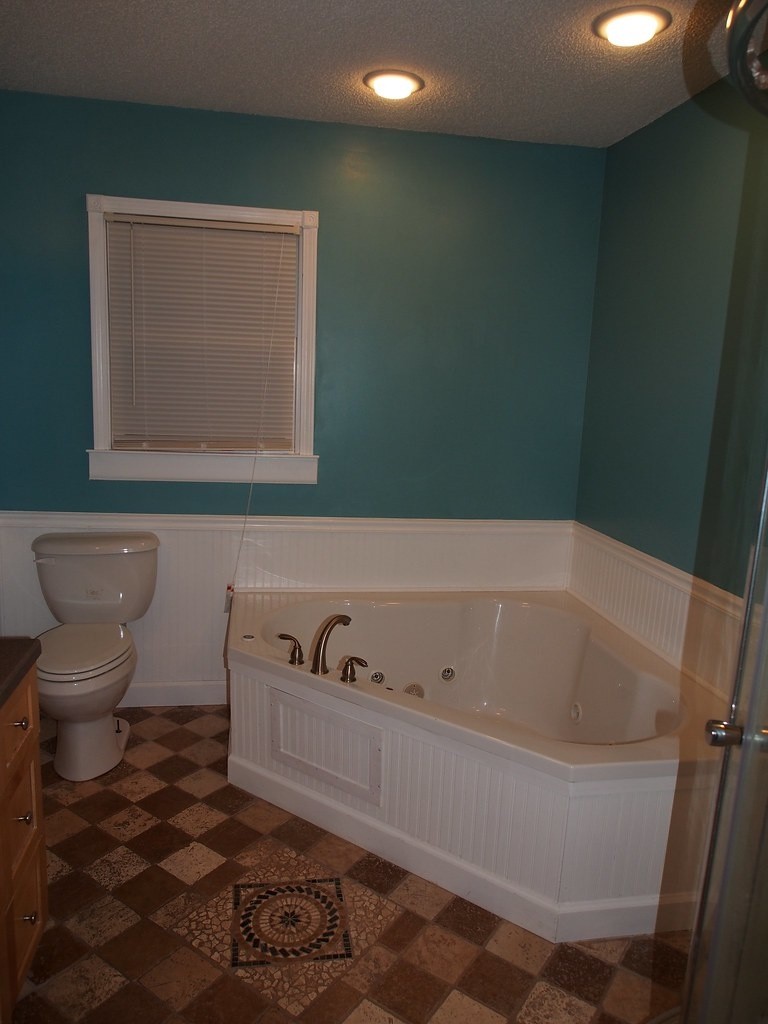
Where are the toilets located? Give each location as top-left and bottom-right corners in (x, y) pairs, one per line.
(28, 531), (162, 781)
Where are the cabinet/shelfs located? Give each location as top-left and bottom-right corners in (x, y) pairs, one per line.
(0, 662), (51, 1024)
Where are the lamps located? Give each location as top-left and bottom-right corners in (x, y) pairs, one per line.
(362, 69), (426, 100)
(592, 4), (673, 48)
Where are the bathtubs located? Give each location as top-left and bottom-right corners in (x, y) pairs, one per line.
(231, 590), (734, 943)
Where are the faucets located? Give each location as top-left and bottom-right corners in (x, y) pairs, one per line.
(309, 613), (353, 675)
